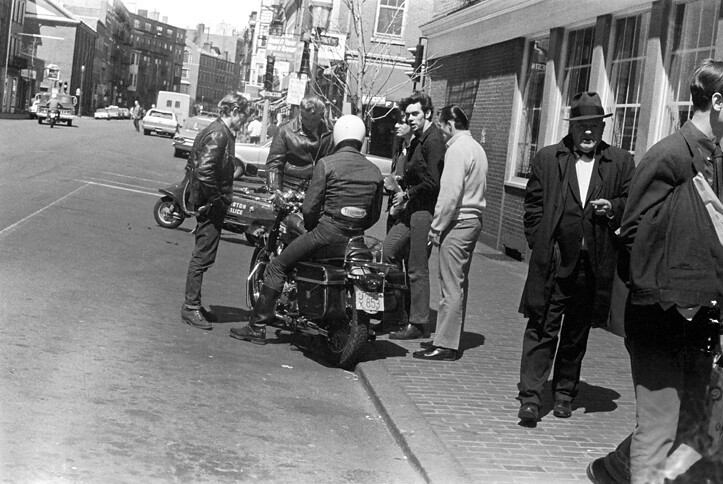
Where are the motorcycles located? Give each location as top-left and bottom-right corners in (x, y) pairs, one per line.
(46, 105), (61, 127)
(153, 166), (284, 246)
(244, 163), (412, 369)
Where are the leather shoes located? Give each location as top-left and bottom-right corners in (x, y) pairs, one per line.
(553, 399), (574, 417)
(418, 340), (435, 351)
(198, 304), (215, 321)
(388, 323), (424, 340)
(180, 306), (214, 331)
(413, 347), (457, 360)
(517, 400), (540, 423)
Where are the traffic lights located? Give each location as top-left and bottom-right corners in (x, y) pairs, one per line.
(64, 82), (67, 91)
(405, 44), (424, 81)
(264, 55), (276, 90)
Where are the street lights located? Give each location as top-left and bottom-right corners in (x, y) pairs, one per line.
(307, 3), (333, 95)
(77, 65), (86, 117)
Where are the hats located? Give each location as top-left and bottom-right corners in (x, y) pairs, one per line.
(562, 91), (616, 121)
(51, 93), (58, 99)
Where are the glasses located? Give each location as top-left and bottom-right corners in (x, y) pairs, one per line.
(240, 109), (250, 119)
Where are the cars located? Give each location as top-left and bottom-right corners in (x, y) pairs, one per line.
(29, 92), (78, 125)
(173, 112), (221, 159)
(234, 136), (396, 194)
(143, 109), (178, 137)
(94, 105), (132, 120)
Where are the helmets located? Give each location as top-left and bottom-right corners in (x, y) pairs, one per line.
(332, 115), (366, 147)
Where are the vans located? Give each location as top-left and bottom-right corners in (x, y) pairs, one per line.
(155, 91), (197, 118)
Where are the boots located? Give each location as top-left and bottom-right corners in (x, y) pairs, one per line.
(229, 284), (282, 345)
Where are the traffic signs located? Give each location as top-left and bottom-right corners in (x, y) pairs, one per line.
(258, 89), (282, 100)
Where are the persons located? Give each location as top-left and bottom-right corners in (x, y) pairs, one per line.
(516, 91), (636, 424)
(373, 92), (446, 340)
(264, 93), (336, 209)
(181, 93), (250, 329)
(247, 116), (262, 145)
(46, 92), (62, 123)
(133, 100), (141, 133)
(267, 119), (279, 140)
(587, 60), (723, 484)
(228, 111), (385, 346)
(412, 105), (488, 360)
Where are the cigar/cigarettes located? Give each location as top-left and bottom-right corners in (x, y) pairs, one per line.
(594, 198), (598, 209)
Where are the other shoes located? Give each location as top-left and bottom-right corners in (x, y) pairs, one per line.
(381, 308), (409, 326)
(586, 453), (623, 484)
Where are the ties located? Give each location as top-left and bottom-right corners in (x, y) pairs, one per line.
(713, 145), (723, 206)
